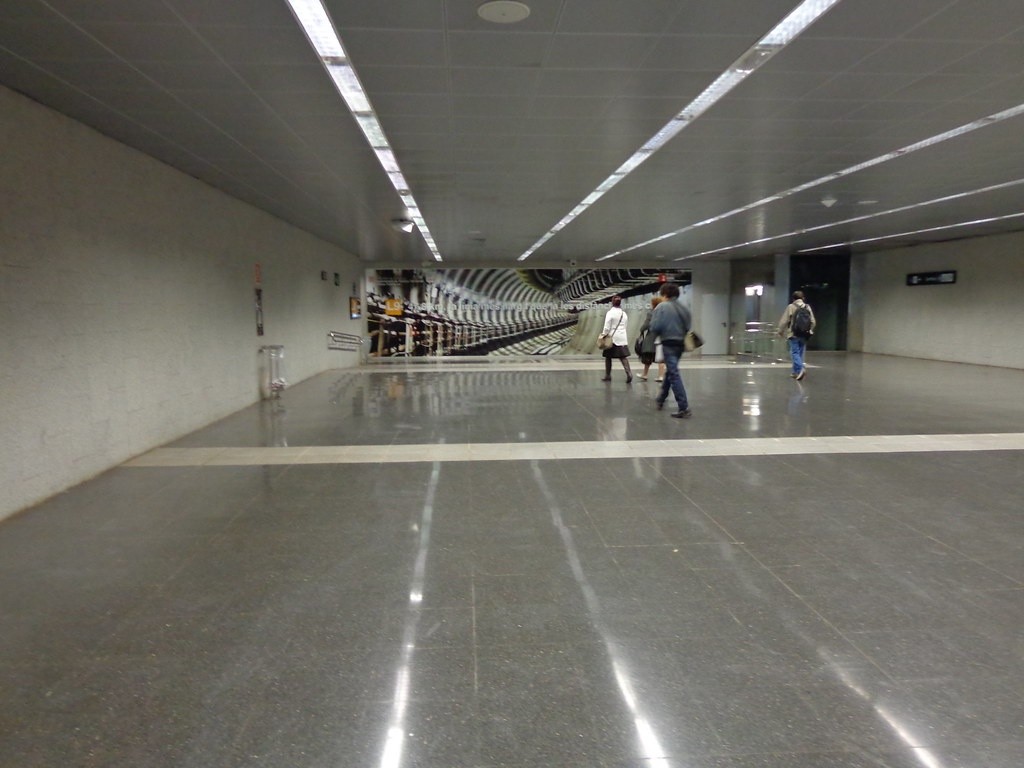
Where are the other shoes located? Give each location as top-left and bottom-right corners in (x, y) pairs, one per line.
(654, 377), (662, 381)
(671, 408), (692, 418)
(601, 375), (612, 381)
(625, 375), (633, 383)
(635, 373), (648, 381)
(796, 369), (806, 380)
(790, 374), (797, 377)
(655, 397), (662, 410)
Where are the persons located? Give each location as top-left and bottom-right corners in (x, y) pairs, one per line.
(636, 298), (664, 381)
(649, 283), (693, 418)
(601, 296), (633, 384)
(778, 290), (816, 380)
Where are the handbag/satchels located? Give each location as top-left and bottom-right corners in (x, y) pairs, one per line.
(635, 331), (644, 355)
(684, 331), (702, 351)
(596, 332), (613, 349)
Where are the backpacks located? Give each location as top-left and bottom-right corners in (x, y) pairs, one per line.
(792, 302), (811, 340)
